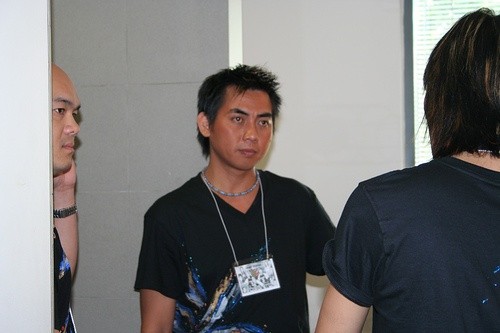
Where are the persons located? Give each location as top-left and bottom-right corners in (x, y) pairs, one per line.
(52, 62), (81, 333)
(132, 64), (337, 333)
(312, 6), (500, 333)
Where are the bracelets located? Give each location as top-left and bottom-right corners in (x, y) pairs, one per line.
(54, 203), (77, 219)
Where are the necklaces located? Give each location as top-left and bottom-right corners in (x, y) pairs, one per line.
(201, 167), (260, 196)
